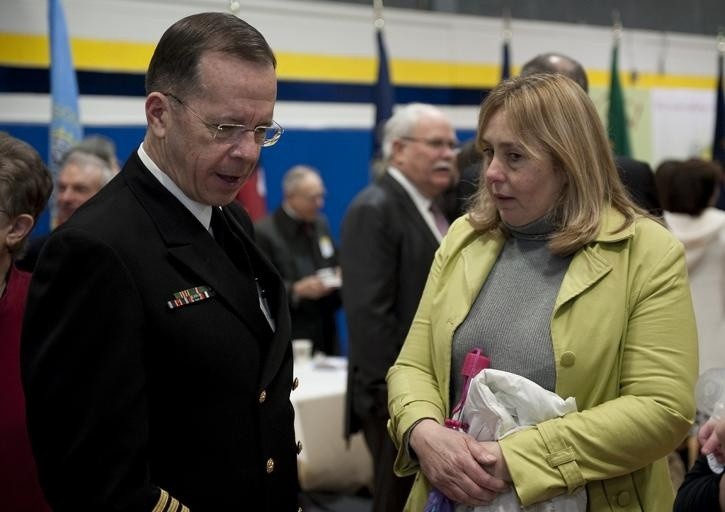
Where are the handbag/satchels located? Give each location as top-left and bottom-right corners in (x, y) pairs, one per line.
(455, 369), (587, 512)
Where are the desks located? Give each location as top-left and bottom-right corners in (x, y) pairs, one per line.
(290, 355), (376, 495)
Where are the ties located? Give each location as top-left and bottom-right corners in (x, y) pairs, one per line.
(210, 206), (280, 329)
(431, 201), (450, 236)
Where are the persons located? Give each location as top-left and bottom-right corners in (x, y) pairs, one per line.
(2, 133), (53, 511)
(21, 9), (305, 512)
(54, 138), (119, 225)
(254, 165), (343, 355)
(342, 57), (725, 512)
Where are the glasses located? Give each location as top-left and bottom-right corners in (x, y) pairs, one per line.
(162, 92), (284, 147)
(402, 136), (455, 150)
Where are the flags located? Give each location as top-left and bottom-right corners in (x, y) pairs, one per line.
(368, 23), (394, 165)
(603, 42), (634, 159)
(713, 48), (725, 213)
(497, 39), (515, 79)
(42, 0), (86, 231)
